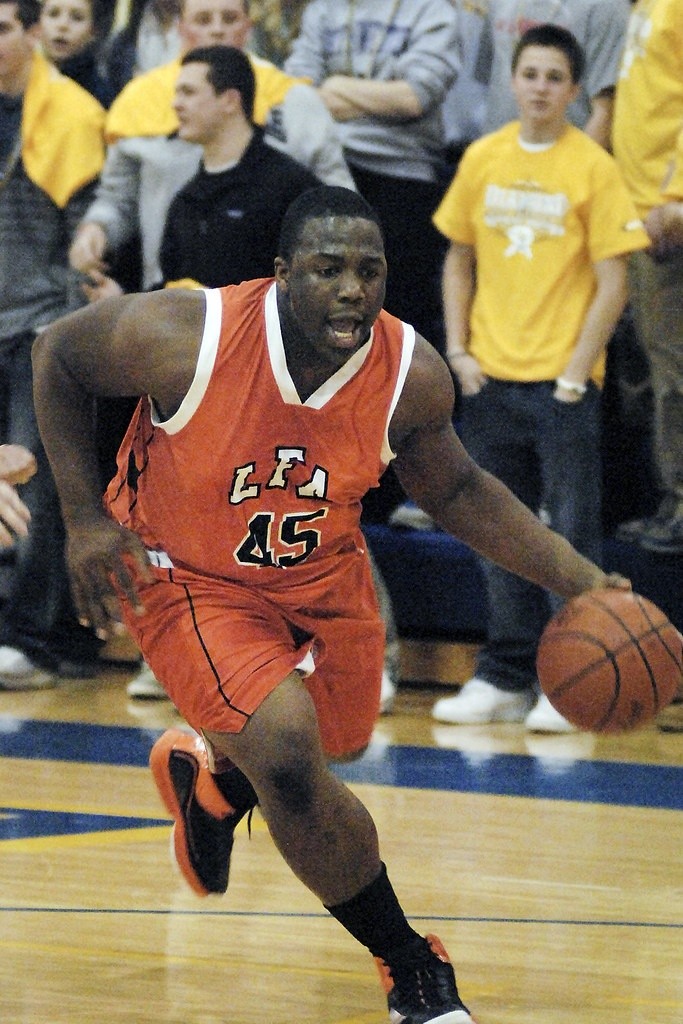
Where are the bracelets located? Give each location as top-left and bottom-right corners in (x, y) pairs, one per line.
(446, 348), (467, 362)
(557, 378), (587, 394)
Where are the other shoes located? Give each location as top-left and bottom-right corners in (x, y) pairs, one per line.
(656, 702), (683, 732)
(379, 671), (395, 713)
(0, 645), (59, 690)
(617, 513), (683, 555)
(390, 500), (437, 532)
(126, 664), (169, 699)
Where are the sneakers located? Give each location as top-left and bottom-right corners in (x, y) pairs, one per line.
(432, 676), (533, 723)
(149, 722), (255, 897)
(527, 686), (575, 732)
(375, 934), (475, 1024)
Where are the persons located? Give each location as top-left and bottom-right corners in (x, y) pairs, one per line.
(430, 19), (651, 737)
(0, 0), (683, 689)
(31, 187), (632, 1024)
(137, 44), (398, 714)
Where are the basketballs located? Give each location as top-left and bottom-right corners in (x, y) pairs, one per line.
(536, 587), (682, 735)
